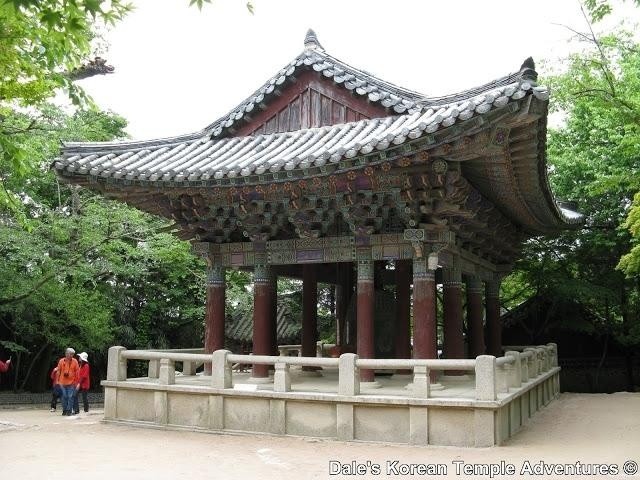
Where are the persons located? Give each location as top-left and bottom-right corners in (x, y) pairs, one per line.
(75, 352), (90, 412)
(50, 357), (63, 412)
(56, 347), (79, 416)
(0, 358), (10, 372)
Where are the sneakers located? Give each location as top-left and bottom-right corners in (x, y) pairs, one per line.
(50, 408), (56, 412)
(62, 410), (79, 416)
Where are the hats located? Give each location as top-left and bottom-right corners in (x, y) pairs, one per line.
(77, 352), (89, 362)
